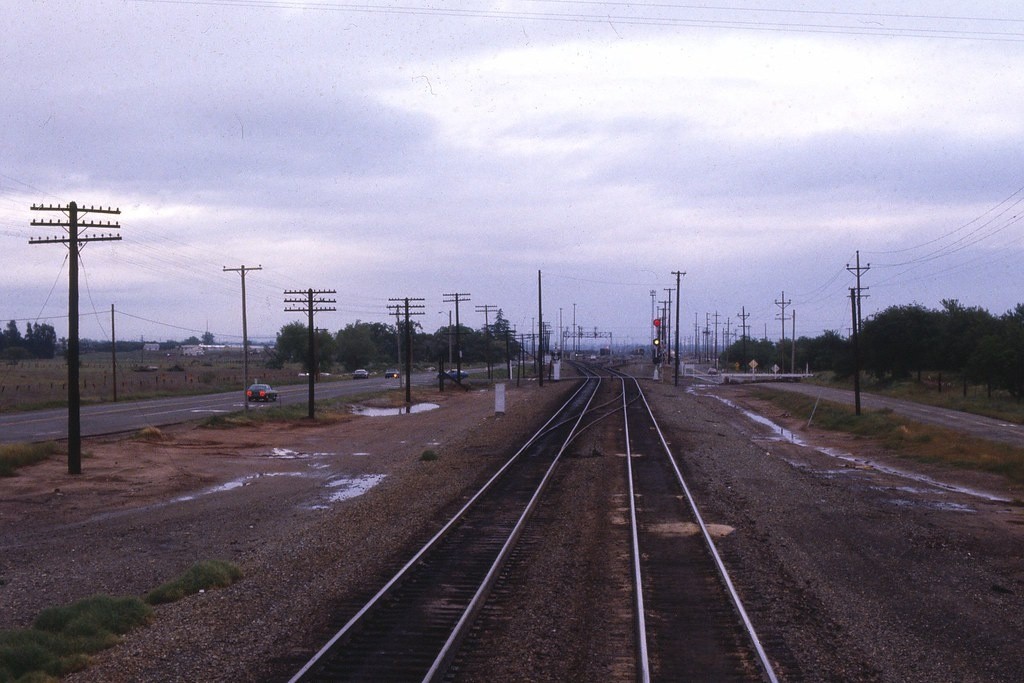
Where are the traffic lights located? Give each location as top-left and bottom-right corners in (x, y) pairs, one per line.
(653, 338), (660, 347)
(653, 319), (661, 328)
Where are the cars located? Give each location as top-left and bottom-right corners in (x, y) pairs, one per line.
(352, 369), (369, 379)
(384, 368), (400, 379)
(708, 368), (717, 374)
(247, 383), (278, 402)
(442, 368), (469, 380)
